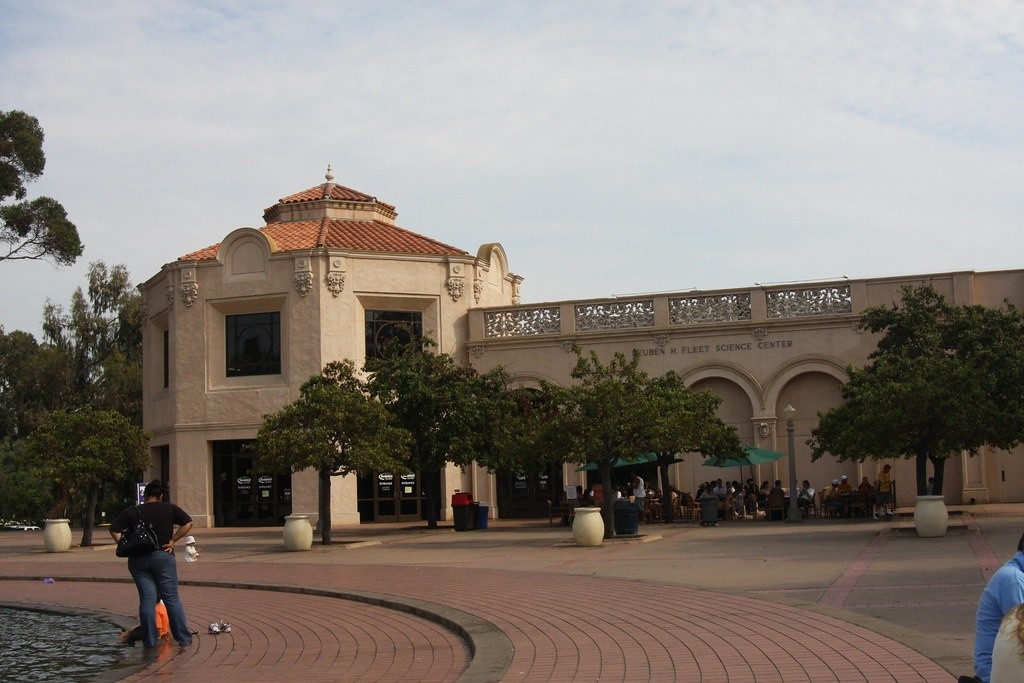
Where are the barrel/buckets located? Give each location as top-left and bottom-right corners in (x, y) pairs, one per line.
(914, 494), (949, 538)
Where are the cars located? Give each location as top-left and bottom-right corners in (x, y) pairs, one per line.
(0, 518), (41, 530)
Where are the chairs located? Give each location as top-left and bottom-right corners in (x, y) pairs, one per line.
(544, 479), (896, 529)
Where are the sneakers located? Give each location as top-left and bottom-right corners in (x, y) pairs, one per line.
(220, 620), (232, 632)
(208, 623), (220, 635)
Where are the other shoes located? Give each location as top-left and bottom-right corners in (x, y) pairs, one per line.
(888, 512), (894, 516)
(873, 513), (878, 521)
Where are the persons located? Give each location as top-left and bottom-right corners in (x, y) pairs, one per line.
(823, 463), (897, 517)
(759, 477), (816, 518)
(126, 593), (169, 646)
(926, 477), (934, 495)
(109, 478), (196, 648)
(973, 532), (1023, 683)
(989, 603), (1024, 683)
(698, 478), (760, 519)
(576, 474), (692, 523)
(184, 535), (199, 562)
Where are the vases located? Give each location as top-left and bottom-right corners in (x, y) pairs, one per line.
(914, 496), (948, 537)
(44, 519), (72, 553)
(285, 516), (313, 552)
(573, 507), (604, 547)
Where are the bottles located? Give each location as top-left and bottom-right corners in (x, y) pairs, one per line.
(573, 506), (604, 547)
(44, 518), (72, 552)
(283, 515), (313, 551)
(43, 578), (54, 584)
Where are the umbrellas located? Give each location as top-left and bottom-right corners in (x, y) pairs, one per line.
(573, 451), (675, 478)
(702, 445), (786, 502)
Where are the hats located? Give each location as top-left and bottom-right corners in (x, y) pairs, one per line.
(185, 535), (195, 545)
(841, 475), (848, 479)
(832, 480), (840, 485)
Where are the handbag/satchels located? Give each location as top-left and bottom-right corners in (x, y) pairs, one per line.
(116, 504), (159, 557)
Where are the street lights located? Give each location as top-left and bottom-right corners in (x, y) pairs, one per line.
(783, 404), (805, 523)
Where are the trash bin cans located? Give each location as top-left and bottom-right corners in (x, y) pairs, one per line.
(700, 497), (718, 527)
(451, 492), (474, 531)
(472, 503), (479, 529)
(613, 501), (640, 537)
(98, 509), (108, 524)
(475, 506), (489, 529)
